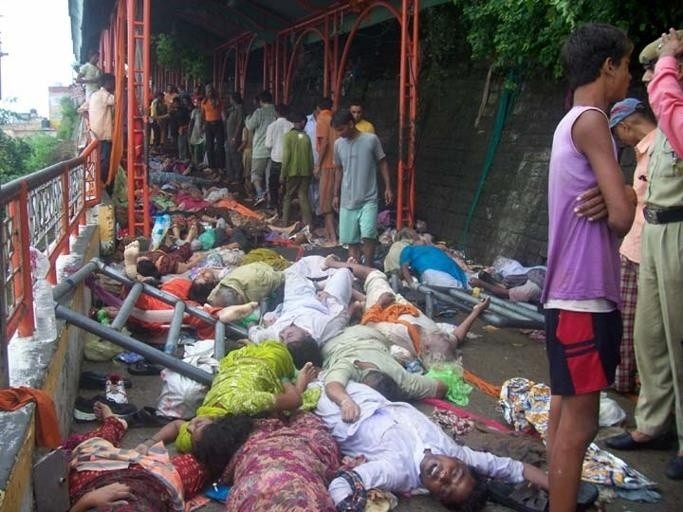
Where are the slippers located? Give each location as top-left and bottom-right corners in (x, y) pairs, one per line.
(486, 476), (547, 512)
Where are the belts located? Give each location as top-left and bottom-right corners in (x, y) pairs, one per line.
(642, 207), (680, 224)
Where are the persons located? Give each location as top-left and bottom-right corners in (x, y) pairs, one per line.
(608, 98), (658, 393)
(67, 50), (549, 512)
(539, 23), (638, 512)
(647, 27), (683, 160)
(607, 30), (683, 480)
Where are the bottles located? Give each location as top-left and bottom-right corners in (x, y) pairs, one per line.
(32, 271), (57, 343)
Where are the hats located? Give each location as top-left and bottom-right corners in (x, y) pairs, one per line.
(639, 30), (683, 65)
(608, 98), (642, 128)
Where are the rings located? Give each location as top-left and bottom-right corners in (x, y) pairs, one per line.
(658, 44), (662, 49)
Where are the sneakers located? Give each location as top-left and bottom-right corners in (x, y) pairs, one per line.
(73, 359), (174, 427)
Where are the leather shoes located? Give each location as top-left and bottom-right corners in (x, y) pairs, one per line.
(664, 453), (682, 480)
(605, 431), (671, 450)
